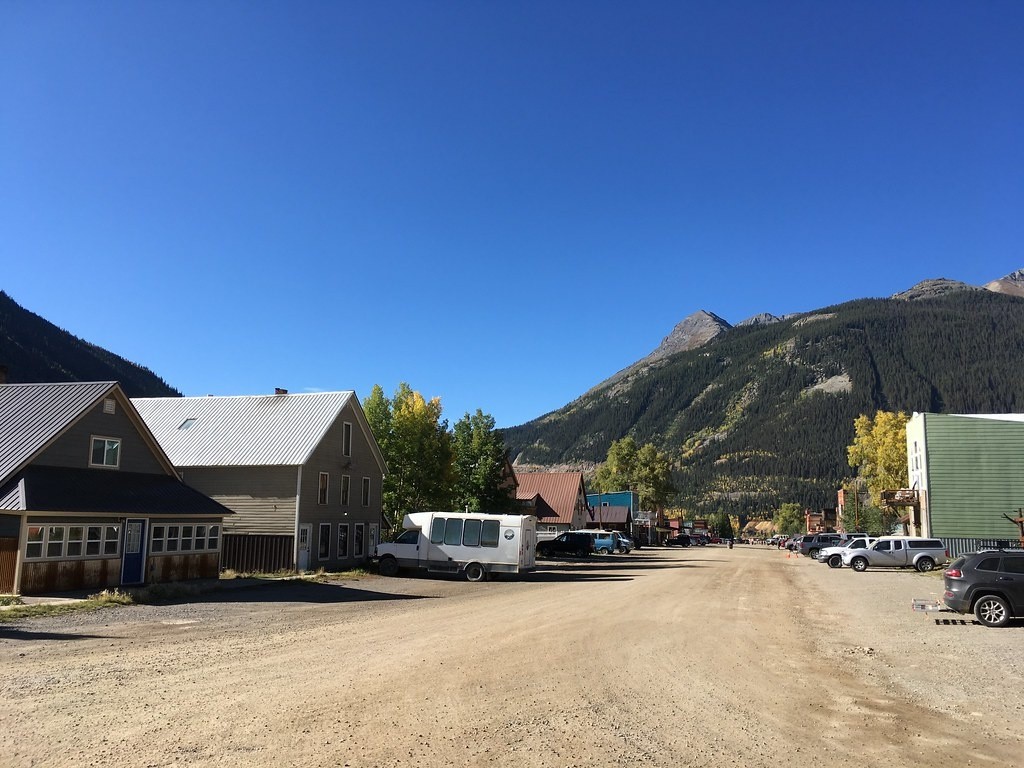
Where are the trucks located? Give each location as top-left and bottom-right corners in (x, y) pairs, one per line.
(374, 512), (538, 582)
(579, 529), (634, 553)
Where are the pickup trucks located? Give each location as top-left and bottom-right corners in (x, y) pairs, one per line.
(843, 534), (949, 572)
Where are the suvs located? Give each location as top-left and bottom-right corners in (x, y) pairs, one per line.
(945, 549), (1024, 627)
(665, 535), (691, 547)
(818, 537), (879, 568)
(689, 535), (722, 546)
(536, 532), (597, 558)
(766, 533), (870, 559)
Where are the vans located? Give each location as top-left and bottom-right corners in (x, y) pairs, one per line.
(560, 529), (617, 554)
